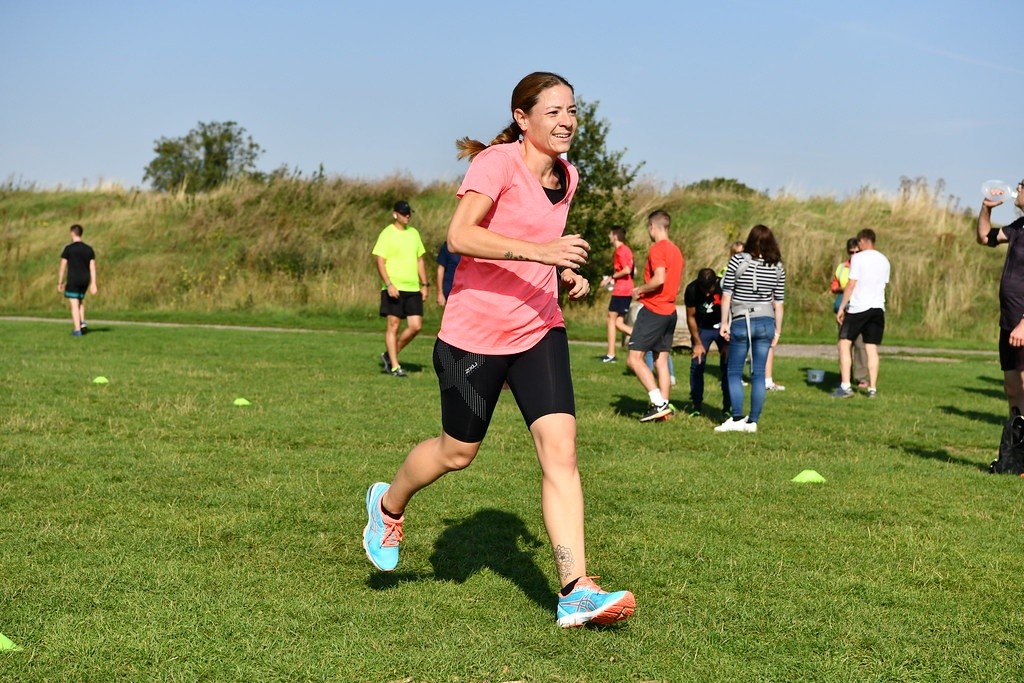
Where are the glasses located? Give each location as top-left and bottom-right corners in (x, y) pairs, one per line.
(849, 251), (860, 255)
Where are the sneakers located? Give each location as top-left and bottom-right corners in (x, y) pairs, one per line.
(363, 481), (405, 571)
(555, 575), (636, 628)
(639, 402), (672, 422)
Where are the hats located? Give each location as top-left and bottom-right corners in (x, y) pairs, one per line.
(394, 201), (415, 214)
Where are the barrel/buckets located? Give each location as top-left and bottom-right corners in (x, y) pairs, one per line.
(808, 369), (824, 382)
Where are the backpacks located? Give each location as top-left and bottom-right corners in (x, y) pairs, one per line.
(988, 405), (1024, 475)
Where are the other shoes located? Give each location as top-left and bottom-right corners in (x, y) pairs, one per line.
(829, 382), (855, 398)
(714, 417), (746, 432)
(391, 366), (407, 377)
(600, 355), (616, 363)
(740, 380), (749, 386)
(689, 401), (704, 420)
(868, 387), (876, 397)
(765, 382), (785, 391)
(856, 378), (867, 388)
(380, 351), (391, 374)
(721, 408), (731, 419)
(79, 321), (88, 329)
(729, 415), (757, 433)
(671, 376), (675, 386)
(70, 329), (82, 336)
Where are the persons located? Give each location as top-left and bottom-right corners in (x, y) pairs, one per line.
(977, 178), (1024, 474)
(372, 201), (461, 377)
(831, 228), (890, 396)
(363, 73), (636, 629)
(600, 209), (786, 432)
(58, 224), (97, 334)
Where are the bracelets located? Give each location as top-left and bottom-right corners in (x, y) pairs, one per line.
(775, 334), (780, 336)
(386, 283), (392, 287)
(422, 283), (429, 286)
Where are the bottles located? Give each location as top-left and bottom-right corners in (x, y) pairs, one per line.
(981, 180), (1018, 201)
(603, 275), (614, 291)
